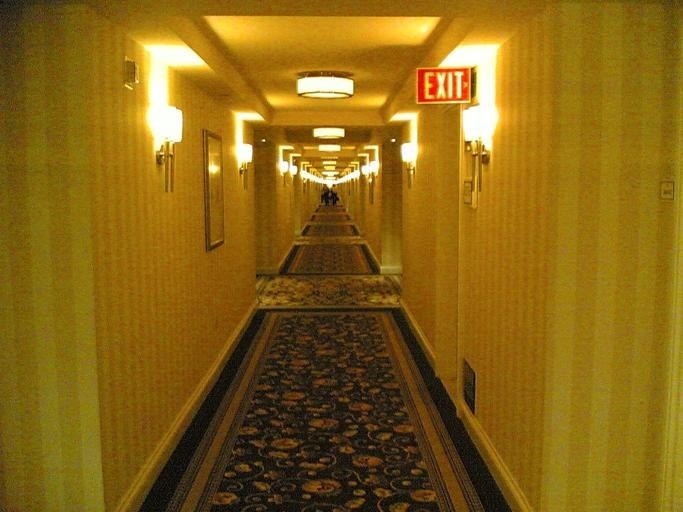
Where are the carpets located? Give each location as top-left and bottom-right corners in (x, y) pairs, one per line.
(300, 205), (359, 237)
(141, 304), (514, 512)
(281, 244), (378, 276)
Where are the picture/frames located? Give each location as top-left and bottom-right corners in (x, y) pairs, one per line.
(202, 128), (225, 253)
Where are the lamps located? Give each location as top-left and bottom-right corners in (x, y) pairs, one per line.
(313, 125), (346, 179)
(462, 105), (489, 193)
(239, 144), (252, 176)
(296, 72), (356, 100)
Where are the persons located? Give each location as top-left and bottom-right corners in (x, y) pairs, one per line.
(323, 184), (339, 208)
